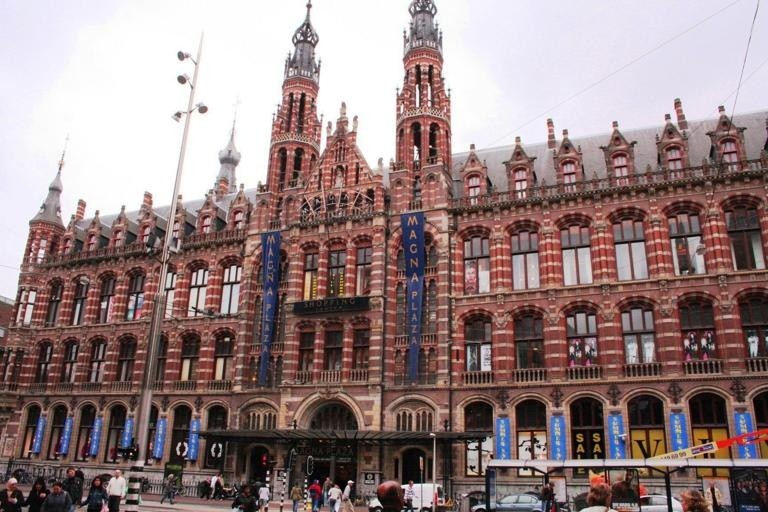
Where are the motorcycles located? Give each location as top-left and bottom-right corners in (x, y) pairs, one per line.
(214, 484), (238, 500)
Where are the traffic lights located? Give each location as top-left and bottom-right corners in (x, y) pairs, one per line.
(306, 456), (315, 475)
(260, 453), (268, 467)
(269, 460), (277, 469)
(126, 447), (136, 460)
(288, 448), (298, 470)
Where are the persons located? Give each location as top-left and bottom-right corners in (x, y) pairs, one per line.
(22, 477), (51, 512)
(160, 473), (175, 504)
(40, 482), (73, 512)
(105, 469), (126, 512)
(0, 478), (25, 512)
(579, 482), (618, 512)
(81, 478), (108, 512)
(63, 467), (83, 512)
(541, 482), (553, 512)
(706, 481), (722, 512)
(201, 471), (418, 512)
(612, 469), (638, 502)
(680, 488), (710, 512)
(735, 480), (768, 504)
(75, 467), (84, 480)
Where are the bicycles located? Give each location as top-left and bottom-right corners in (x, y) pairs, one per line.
(444, 490), (464, 512)
(162, 482), (187, 497)
(0, 471), (65, 488)
(350, 489), (374, 507)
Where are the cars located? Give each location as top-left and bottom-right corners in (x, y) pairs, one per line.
(471, 492), (553, 511)
(613, 495), (685, 511)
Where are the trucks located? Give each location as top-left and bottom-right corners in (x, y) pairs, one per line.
(366, 482), (446, 511)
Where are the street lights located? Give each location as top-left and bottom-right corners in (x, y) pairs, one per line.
(428, 431), (436, 511)
(112, 28), (211, 511)
